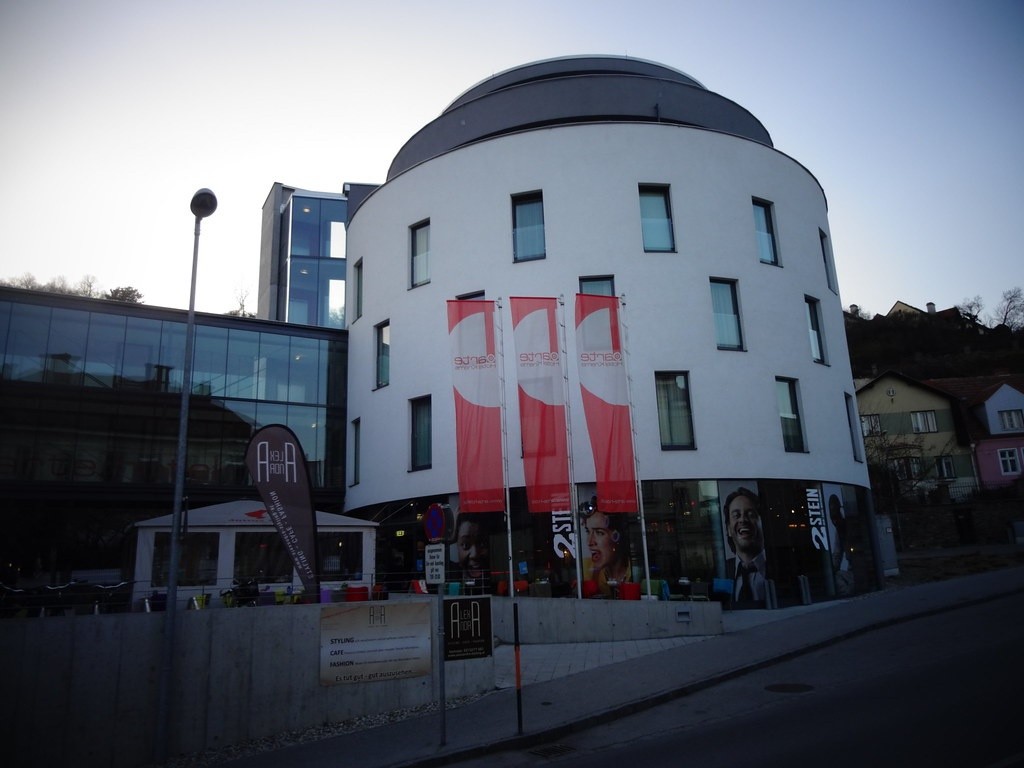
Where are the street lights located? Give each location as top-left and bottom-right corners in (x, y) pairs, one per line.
(144, 187), (220, 768)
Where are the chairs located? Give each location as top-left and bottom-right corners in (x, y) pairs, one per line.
(662, 578), (686, 601)
(686, 581), (710, 602)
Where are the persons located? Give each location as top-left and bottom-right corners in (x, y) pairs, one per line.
(828, 496), (871, 598)
(577, 497), (642, 601)
(718, 488), (796, 610)
(445, 512), (496, 597)
